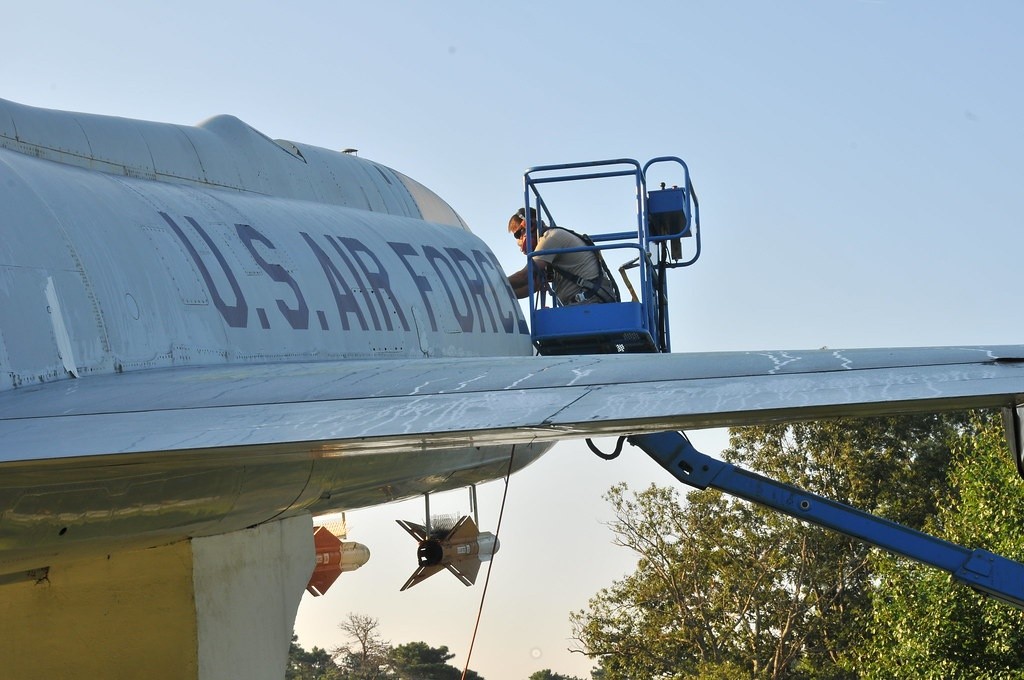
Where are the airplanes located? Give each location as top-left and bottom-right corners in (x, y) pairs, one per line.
(0, 98), (1024, 592)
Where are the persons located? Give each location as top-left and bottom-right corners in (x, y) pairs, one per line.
(506, 207), (617, 307)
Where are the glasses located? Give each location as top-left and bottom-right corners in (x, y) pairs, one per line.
(514, 226), (524, 240)
